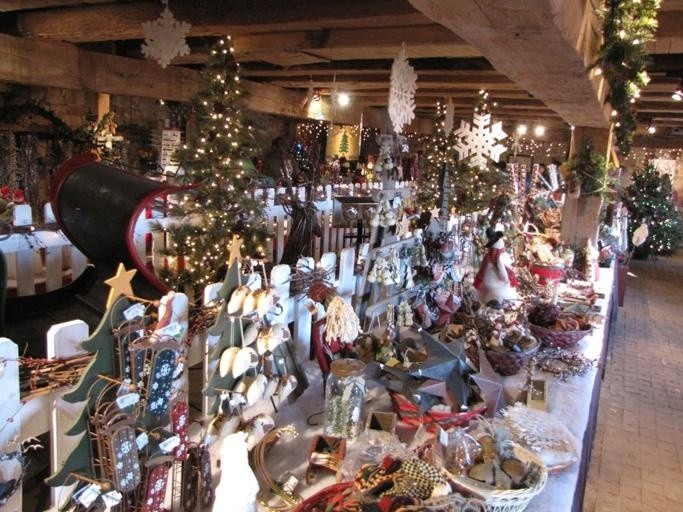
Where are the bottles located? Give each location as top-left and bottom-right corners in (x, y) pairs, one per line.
(323, 358), (366, 446)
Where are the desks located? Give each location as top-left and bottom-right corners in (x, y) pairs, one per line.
(251, 253), (620, 511)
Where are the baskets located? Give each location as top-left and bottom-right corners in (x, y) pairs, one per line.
(427, 439), (549, 512)
(463, 326), (542, 377)
(528, 311), (593, 350)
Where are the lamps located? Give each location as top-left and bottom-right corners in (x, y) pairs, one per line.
(674, 81), (683, 96)
